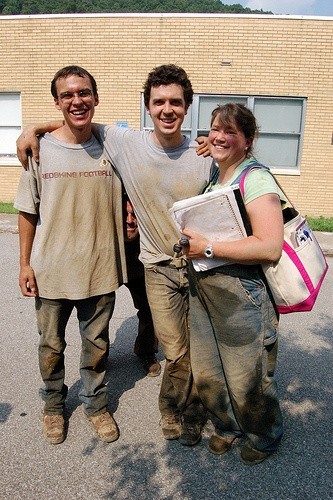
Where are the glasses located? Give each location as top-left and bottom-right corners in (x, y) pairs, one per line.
(57, 89), (95, 104)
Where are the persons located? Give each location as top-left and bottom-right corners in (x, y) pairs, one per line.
(110, 188), (161, 377)
(19, 64), (213, 445)
(174, 103), (287, 463)
(16, 63), (220, 446)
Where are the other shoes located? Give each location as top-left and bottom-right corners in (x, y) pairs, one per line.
(239, 444), (267, 464)
(207, 430), (235, 455)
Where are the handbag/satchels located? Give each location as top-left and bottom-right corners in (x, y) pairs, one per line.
(241, 164), (328, 313)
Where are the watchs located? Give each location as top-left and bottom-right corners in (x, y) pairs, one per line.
(204, 240), (214, 259)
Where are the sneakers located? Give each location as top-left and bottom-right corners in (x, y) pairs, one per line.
(159, 412), (180, 439)
(179, 415), (202, 444)
(83, 409), (119, 442)
(137, 353), (161, 376)
(42, 413), (65, 444)
